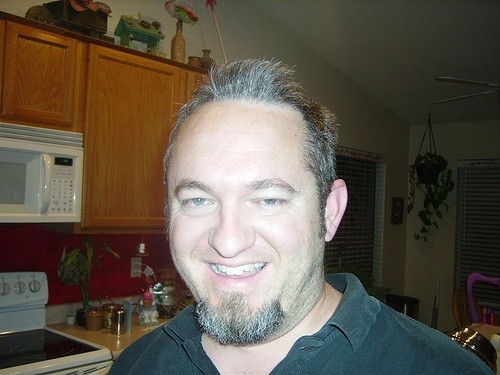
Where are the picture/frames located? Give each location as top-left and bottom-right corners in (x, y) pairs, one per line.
(391, 198), (403, 224)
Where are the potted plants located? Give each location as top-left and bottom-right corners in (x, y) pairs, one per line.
(406, 151), (454, 243)
(58, 239), (106, 327)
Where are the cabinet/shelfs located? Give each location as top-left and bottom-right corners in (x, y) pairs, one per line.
(0, 9), (212, 235)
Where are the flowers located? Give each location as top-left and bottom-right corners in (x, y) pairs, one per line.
(165, 1), (198, 26)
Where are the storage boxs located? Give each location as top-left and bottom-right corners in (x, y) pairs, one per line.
(43, 0), (107, 33)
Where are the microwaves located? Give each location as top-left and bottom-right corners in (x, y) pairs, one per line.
(0, 123), (85, 223)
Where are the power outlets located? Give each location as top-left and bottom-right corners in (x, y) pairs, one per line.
(131, 257), (142, 277)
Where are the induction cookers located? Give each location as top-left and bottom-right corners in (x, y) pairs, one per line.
(0, 270), (114, 375)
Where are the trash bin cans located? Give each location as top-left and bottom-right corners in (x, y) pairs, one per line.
(386, 294), (420, 320)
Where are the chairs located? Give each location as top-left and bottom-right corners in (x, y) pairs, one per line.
(453, 288), (481, 328)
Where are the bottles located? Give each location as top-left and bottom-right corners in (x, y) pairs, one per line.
(111, 309), (125, 335)
(171, 20), (186, 64)
(187, 56), (202, 67)
(103, 303), (121, 329)
(201, 48), (216, 70)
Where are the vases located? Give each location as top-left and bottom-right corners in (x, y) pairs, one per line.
(171, 22), (185, 63)
(202, 49), (215, 69)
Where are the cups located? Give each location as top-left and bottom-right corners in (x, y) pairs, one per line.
(85, 311), (104, 331)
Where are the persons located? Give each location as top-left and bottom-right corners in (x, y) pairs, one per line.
(104, 58), (494, 375)
